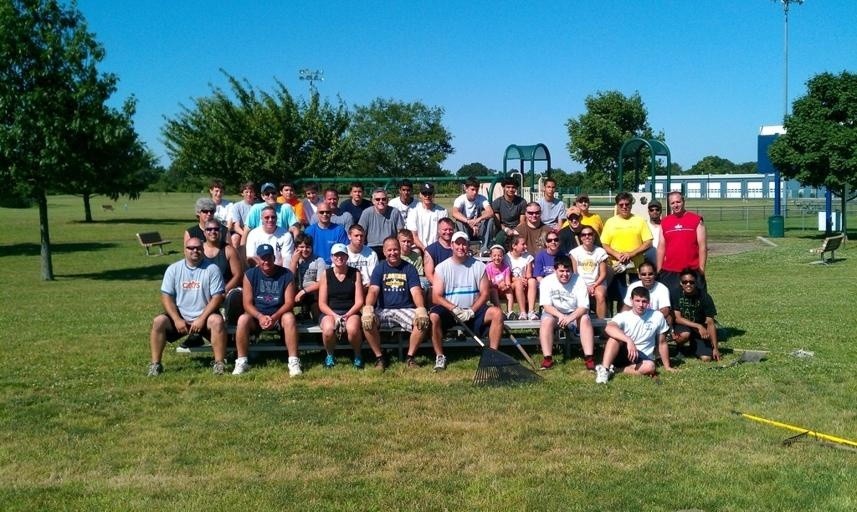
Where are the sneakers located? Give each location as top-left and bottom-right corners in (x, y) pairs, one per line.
(232, 361), (249, 375)
(433, 358), (447, 370)
(585, 360), (595, 370)
(518, 313), (528, 320)
(480, 248), (491, 257)
(595, 364), (614, 376)
(538, 360), (555, 371)
(374, 357), (387, 373)
(502, 310), (507, 320)
(287, 360), (302, 378)
(506, 311), (518, 320)
(213, 362), (225, 376)
(596, 367), (610, 385)
(325, 355), (335, 367)
(180, 334), (206, 348)
(354, 355), (364, 369)
(406, 358), (420, 369)
(147, 364), (160, 378)
(528, 312), (539, 320)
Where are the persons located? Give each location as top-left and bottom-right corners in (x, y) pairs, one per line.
(513, 201), (553, 267)
(451, 175), (496, 258)
(360, 235), (430, 373)
(594, 286), (679, 386)
(575, 192), (604, 249)
(147, 236), (228, 377)
(540, 254), (597, 371)
(357, 188), (405, 261)
(276, 181), (307, 227)
(294, 202), (351, 323)
(232, 184), (261, 250)
(180, 219), (244, 349)
(568, 226), (610, 341)
(183, 197), (232, 257)
(387, 179), (420, 231)
(303, 200), (351, 271)
(557, 206), (603, 256)
(486, 244), (518, 321)
(246, 207), (295, 271)
(309, 186), (354, 234)
(342, 223), (379, 304)
(618, 260), (674, 336)
(302, 180), (323, 219)
(600, 191), (654, 314)
(533, 231), (581, 341)
(644, 199), (662, 269)
(428, 230), (504, 374)
(491, 176), (528, 252)
(670, 267), (722, 363)
(317, 242), (364, 369)
(289, 230), (326, 324)
(339, 181), (374, 225)
(208, 179), (235, 227)
(504, 234), (539, 321)
(396, 228), (429, 303)
(535, 177), (567, 233)
(424, 217), (454, 286)
(406, 183), (449, 256)
(231, 243), (303, 379)
(656, 191), (708, 294)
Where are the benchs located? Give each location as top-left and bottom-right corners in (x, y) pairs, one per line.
(136, 231), (172, 255)
(808, 235), (843, 263)
(173, 312), (613, 355)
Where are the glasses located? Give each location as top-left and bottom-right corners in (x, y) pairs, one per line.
(318, 210), (331, 215)
(373, 197), (387, 201)
(582, 231), (593, 238)
(641, 272), (653, 276)
(617, 203), (630, 207)
(184, 247), (203, 250)
(202, 211), (213, 213)
(262, 191), (276, 195)
(568, 215), (580, 220)
(205, 227), (220, 231)
(682, 280), (696, 284)
(545, 237), (559, 242)
(527, 211), (540, 215)
(650, 208), (659, 211)
(263, 215), (276, 219)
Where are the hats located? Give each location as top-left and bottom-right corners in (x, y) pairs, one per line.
(451, 232), (470, 242)
(261, 183), (275, 192)
(330, 244), (349, 255)
(256, 245), (274, 256)
(648, 199), (662, 209)
(566, 205), (580, 218)
(195, 197), (216, 213)
(575, 192), (589, 201)
(419, 181), (434, 190)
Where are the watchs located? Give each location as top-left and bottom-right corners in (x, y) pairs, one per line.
(465, 216), (472, 224)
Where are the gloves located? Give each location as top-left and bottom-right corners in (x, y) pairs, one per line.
(458, 309), (475, 324)
(450, 307), (464, 323)
(613, 258), (636, 274)
(334, 316), (345, 341)
(416, 307), (429, 330)
(362, 306), (377, 330)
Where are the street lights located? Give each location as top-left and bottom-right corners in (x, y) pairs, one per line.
(774, 0), (803, 116)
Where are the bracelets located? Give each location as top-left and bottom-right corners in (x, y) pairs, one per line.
(254, 310), (261, 319)
